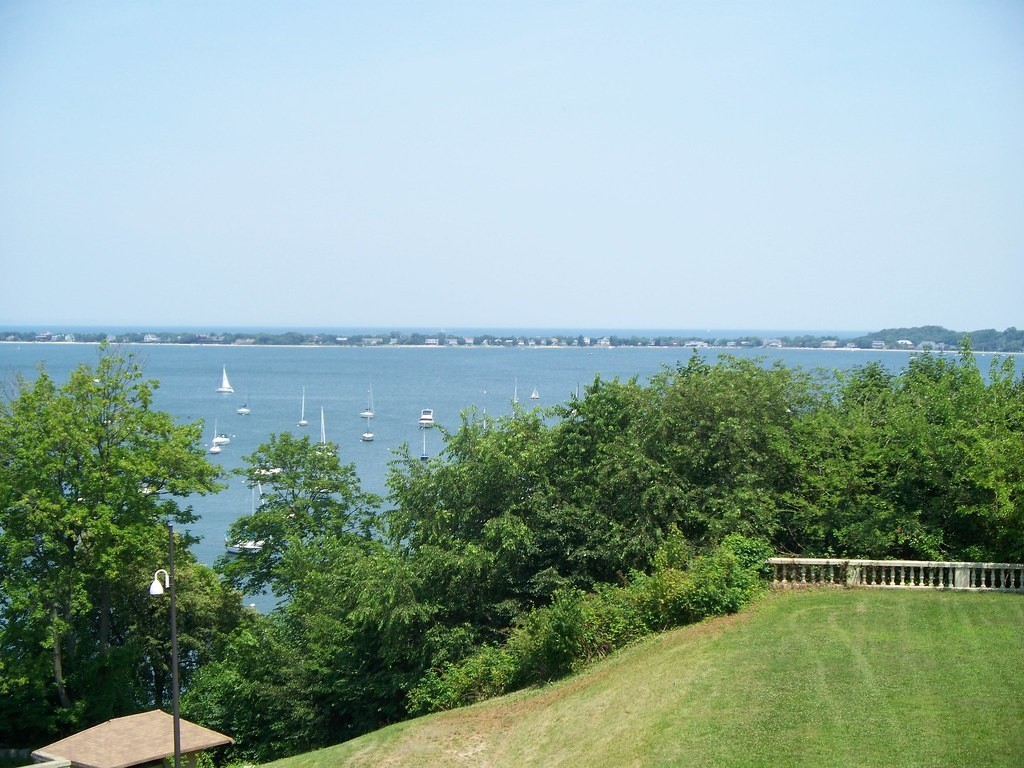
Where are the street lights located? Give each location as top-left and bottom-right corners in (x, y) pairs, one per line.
(149, 524), (181, 768)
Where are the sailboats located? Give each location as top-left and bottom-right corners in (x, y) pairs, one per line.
(362, 415), (375, 441)
(361, 383), (375, 418)
(530, 387), (539, 400)
(224, 462), (272, 553)
(217, 365), (234, 393)
(298, 386), (308, 425)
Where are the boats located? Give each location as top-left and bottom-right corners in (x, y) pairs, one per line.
(418, 409), (434, 428)
(209, 419), (230, 454)
(237, 408), (251, 416)
